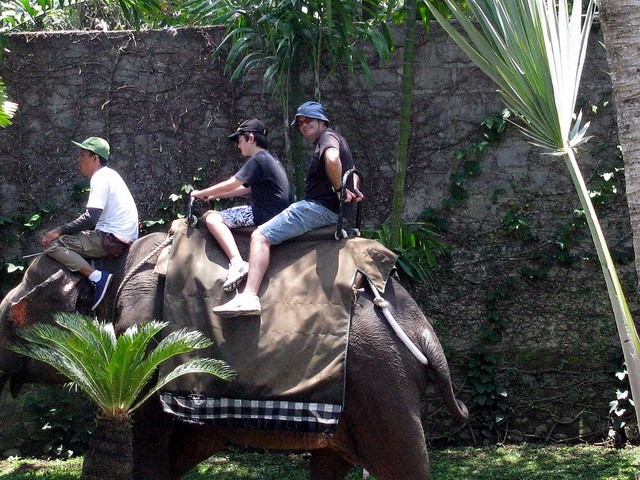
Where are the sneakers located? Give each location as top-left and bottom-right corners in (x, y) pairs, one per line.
(223, 261), (249, 292)
(90, 271), (113, 311)
(213, 289), (261, 319)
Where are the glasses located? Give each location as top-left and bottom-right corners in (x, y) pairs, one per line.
(297, 116), (313, 127)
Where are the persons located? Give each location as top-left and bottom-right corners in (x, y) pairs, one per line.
(212, 100), (365, 320)
(190, 117), (290, 292)
(41, 136), (138, 311)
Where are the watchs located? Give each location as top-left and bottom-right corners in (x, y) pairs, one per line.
(331, 186), (342, 193)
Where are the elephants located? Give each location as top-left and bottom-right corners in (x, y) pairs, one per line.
(0, 224), (471, 480)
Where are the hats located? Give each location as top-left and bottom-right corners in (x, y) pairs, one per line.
(227, 118), (268, 142)
(71, 137), (110, 160)
(291, 101), (330, 126)
